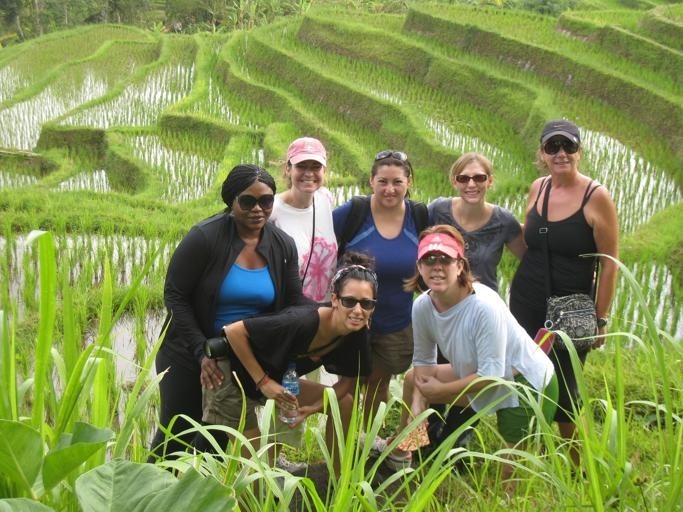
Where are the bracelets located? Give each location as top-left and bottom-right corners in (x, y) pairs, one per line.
(254, 373), (269, 390)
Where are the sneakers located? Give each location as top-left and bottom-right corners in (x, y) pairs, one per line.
(353, 430), (411, 472)
(272, 454), (307, 475)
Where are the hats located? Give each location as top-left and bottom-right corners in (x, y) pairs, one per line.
(539, 121), (580, 146)
(285, 137), (327, 169)
(416, 231), (464, 264)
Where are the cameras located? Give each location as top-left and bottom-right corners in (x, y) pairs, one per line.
(205, 322), (230, 359)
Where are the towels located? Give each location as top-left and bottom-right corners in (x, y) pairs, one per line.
(271, 408), (306, 448)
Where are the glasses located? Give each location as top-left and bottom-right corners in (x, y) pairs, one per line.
(542, 139), (577, 154)
(374, 150), (408, 162)
(336, 294), (376, 310)
(419, 253), (453, 267)
(455, 174), (488, 184)
(235, 194), (273, 211)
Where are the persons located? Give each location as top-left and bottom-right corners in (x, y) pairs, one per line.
(332, 150), (431, 433)
(200, 252), (379, 512)
(509, 119), (619, 479)
(353, 224), (559, 500)
(425, 153), (528, 474)
(266, 136), (339, 304)
(147, 163), (303, 479)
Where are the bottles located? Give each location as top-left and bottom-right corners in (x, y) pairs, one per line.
(280, 362), (299, 423)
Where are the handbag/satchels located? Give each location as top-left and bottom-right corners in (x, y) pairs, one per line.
(547, 293), (600, 350)
